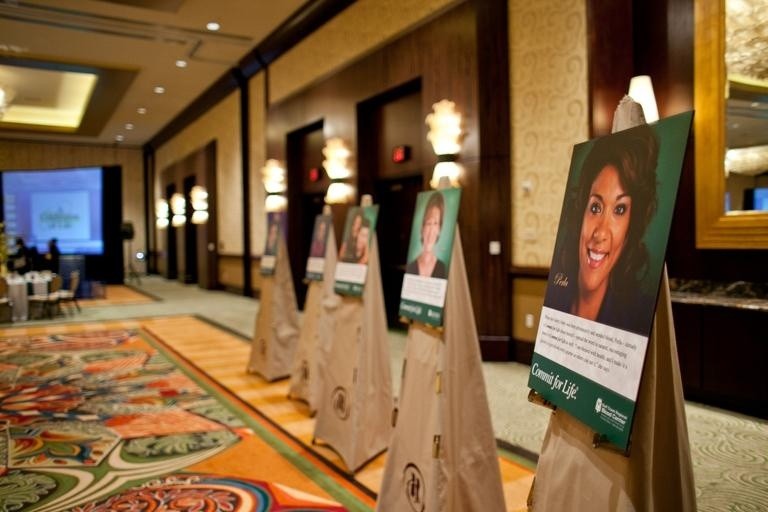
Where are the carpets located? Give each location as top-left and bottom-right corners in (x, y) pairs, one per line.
(60, 282), (164, 311)
(0, 313), (542, 512)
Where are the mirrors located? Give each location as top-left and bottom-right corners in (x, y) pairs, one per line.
(693, 0), (768, 249)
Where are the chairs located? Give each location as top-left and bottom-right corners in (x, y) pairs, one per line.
(1, 266), (83, 322)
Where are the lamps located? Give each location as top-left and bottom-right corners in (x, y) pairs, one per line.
(259, 156), (288, 211)
(321, 138), (355, 206)
(423, 99), (465, 192)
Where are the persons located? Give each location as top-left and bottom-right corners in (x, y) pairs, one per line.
(7, 235), (63, 275)
(310, 217), (327, 258)
(348, 220), (372, 264)
(543, 124), (659, 338)
(407, 190), (448, 280)
(338, 208), (368, 265)
(264, 222), (281, 257)
